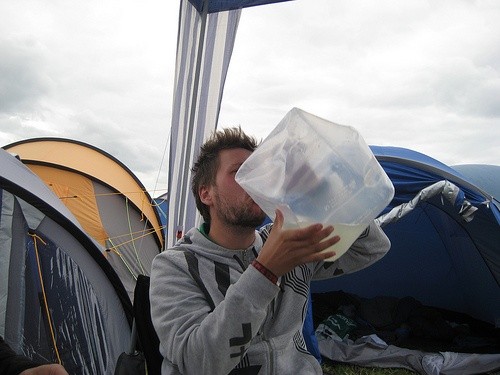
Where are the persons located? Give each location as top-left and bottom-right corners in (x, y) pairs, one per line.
(146, 124), (392, 375)
(0, 334), (70, 375)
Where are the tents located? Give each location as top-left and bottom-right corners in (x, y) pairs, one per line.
(235, 141), (500, 375)
(1, 135), (167, 309)
(0, 145), (136, 375)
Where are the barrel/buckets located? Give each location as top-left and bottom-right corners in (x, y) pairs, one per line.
(234, 108), (395, 263)
